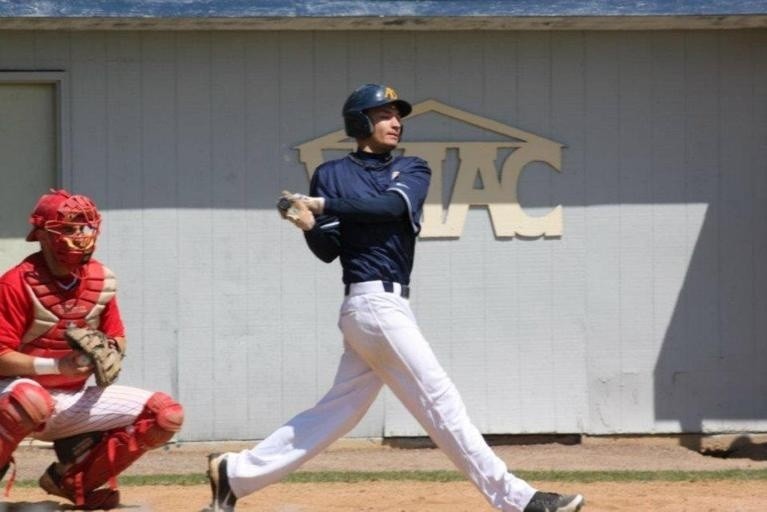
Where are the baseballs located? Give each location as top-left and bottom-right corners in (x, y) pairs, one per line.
(75, 354), (91, 371)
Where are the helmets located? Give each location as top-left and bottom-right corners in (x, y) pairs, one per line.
(343, 84), (412, 143)
(26, 187), (101, 279)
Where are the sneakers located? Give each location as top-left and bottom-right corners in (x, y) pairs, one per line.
(524, 493), (583, 512)
(40, 462), (119, 509)
(207, 453), (236, 512)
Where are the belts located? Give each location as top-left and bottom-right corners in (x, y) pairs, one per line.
(345, 281), (409, 298)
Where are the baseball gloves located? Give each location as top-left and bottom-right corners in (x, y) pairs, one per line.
(65, 326), (123, 387)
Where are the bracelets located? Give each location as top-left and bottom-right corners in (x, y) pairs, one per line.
(32, 356), (63, 377)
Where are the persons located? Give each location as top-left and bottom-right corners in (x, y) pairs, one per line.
(203, 81), (584, 511)
(0, 189), (188, 511)
